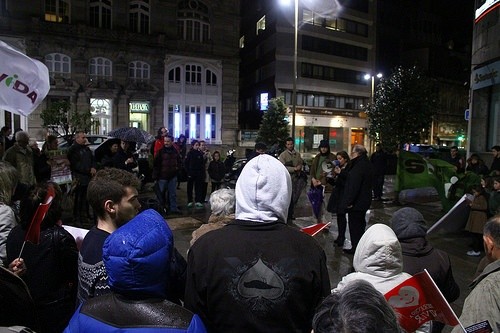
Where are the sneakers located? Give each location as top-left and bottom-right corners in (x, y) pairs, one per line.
(171, 210), (183, 214)
(467, 250), (481, 256)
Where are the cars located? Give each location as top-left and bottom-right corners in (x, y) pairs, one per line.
(58, 135), (113, 152)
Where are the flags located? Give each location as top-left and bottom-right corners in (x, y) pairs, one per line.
(382, 271), (459, 333)
(26, 184), (56, 245)
(300, 222), (328, 236)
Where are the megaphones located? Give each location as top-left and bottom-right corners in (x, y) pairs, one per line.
(322, 159), (341, 173)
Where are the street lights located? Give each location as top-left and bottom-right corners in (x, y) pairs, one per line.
(278, 0), (299, 150)
(362, 68), (383, 157)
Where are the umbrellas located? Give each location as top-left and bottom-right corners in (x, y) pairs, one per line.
(107, 126), (156, 144)
(306, 186), (323, 222)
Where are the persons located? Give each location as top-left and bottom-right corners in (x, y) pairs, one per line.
(329, 224), (433, 333)
(64, 208), (206, 333)
(311, 279), (402, 333)
(389, 207), (460, 333)
(0, 258), (38, 333)
(452, 215), (500, 333)
(0, 125), (500, 265)
(73, 167), (143, 313)
(183, 154), (332, 333)
(5, 180), (78, 333)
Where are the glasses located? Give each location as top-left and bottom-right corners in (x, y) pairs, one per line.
(351, 152), (356, 155)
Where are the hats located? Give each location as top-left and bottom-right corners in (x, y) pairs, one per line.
(319, 140), (330, 147)
(389, 207), (427, 238)
(468, 154), (483, 161)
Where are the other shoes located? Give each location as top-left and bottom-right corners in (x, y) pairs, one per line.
(187, 202), (193, 207)
(343, 247), (356, 254)
(334, 237), (344, 247)
(195, 202), (203, 209)
